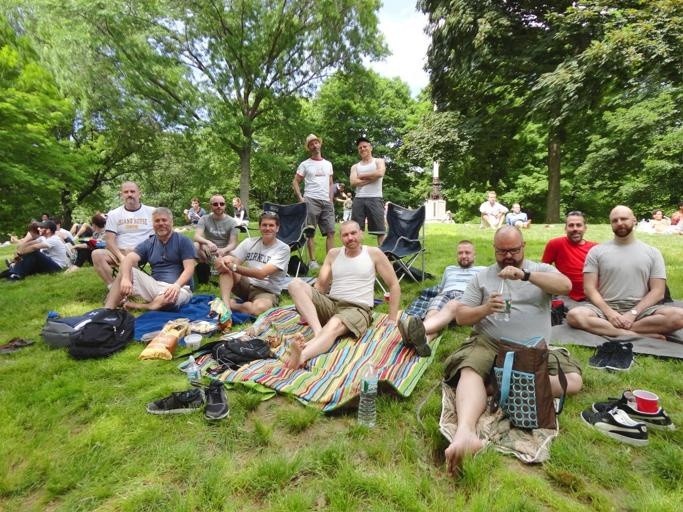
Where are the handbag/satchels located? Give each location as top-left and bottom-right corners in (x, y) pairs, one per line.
(42, 308), (135, 357)
(493, 335), (557, 430)
(212, 339), (269, 367)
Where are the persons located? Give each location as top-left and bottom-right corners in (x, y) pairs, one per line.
(92, 182), (293, 317)
(1, 212), (107, 280)
(349, 137), (386, 247)
(479, 191), (532, 229)
(399, 206), (683, 480)
(293, 133), (335, 269)
(288, 180), (400, 369)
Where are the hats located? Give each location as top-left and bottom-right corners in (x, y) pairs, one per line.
(356, 136), (369, 144)
(305, 133), (323, 151)
(37, 220), (57, 231)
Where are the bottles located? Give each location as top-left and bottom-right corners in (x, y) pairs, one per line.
(494, 276), (512, 322)
(356, 358), (378, 424)
(208, 251), (219, 274)
(187, 356), (201, 388)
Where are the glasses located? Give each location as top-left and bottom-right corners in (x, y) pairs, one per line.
(209, 202), (225, 207)
(568, 211), (583, 216)
(494, 244), (522, 257)
(261, 210), (278, 217)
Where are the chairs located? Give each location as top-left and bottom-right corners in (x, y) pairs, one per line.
(356, 198), (427, 291)
(257, 200), (314, 278)
(96, 233), (155, 285)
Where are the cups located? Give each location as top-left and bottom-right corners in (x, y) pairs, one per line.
(631, 388), (660, 414)
(383, 293), (389, 301)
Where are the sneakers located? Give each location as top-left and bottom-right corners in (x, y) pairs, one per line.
(146, 388), (204, 415)
(589, 341), (635, 372)
(204, 379), (230, 424)
(397, 311), (431, 357)
(580, 390), (675, 448)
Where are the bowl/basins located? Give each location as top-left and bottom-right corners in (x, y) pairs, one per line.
(184, 334), (202, 352)
